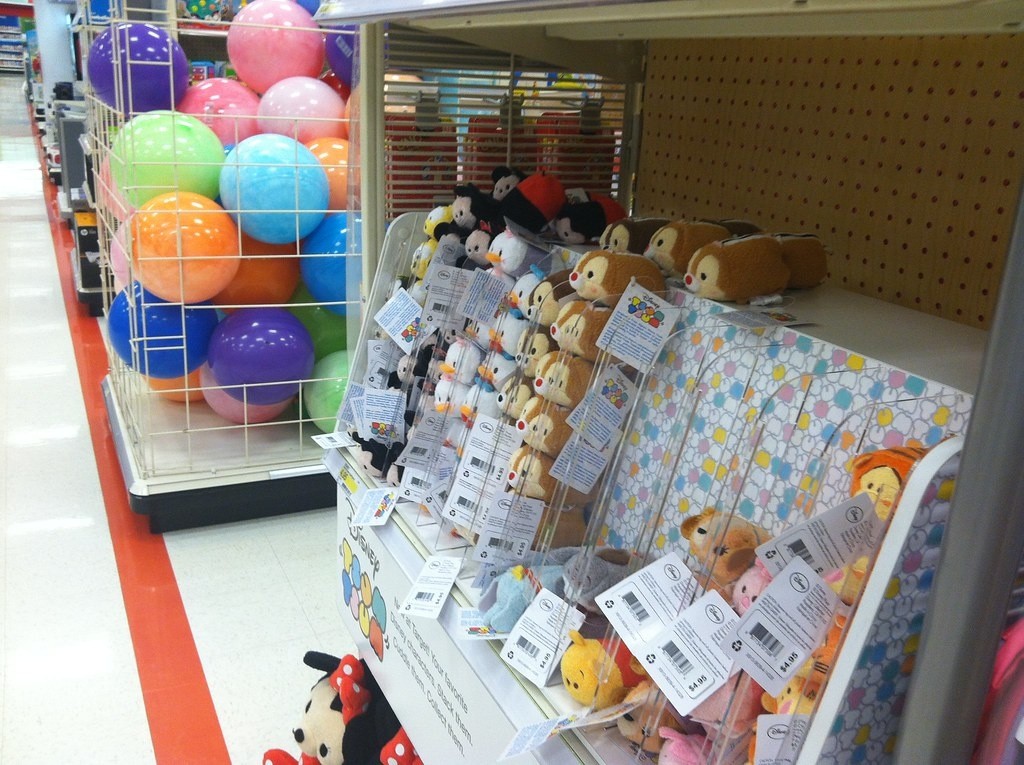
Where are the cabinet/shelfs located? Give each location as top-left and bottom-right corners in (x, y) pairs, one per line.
(263, 0), (1024, 765)
(0, 0), (454, 317)
